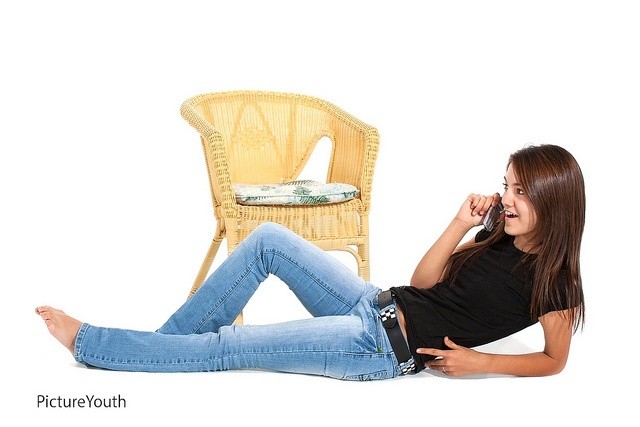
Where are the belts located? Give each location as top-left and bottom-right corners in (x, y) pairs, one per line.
(378, 290), (415, 374)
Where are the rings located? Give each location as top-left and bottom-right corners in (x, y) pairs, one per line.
(442, 366), (447, 374)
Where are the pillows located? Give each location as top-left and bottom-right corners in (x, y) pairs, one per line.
(229, 180), (360, 206)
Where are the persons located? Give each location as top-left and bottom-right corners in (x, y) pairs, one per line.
(34, 145), (586, 382)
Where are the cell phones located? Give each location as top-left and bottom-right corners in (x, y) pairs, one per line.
(481, 197), (503, 232)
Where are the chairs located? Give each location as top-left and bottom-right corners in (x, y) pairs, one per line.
(180, 90), (380, 325)
